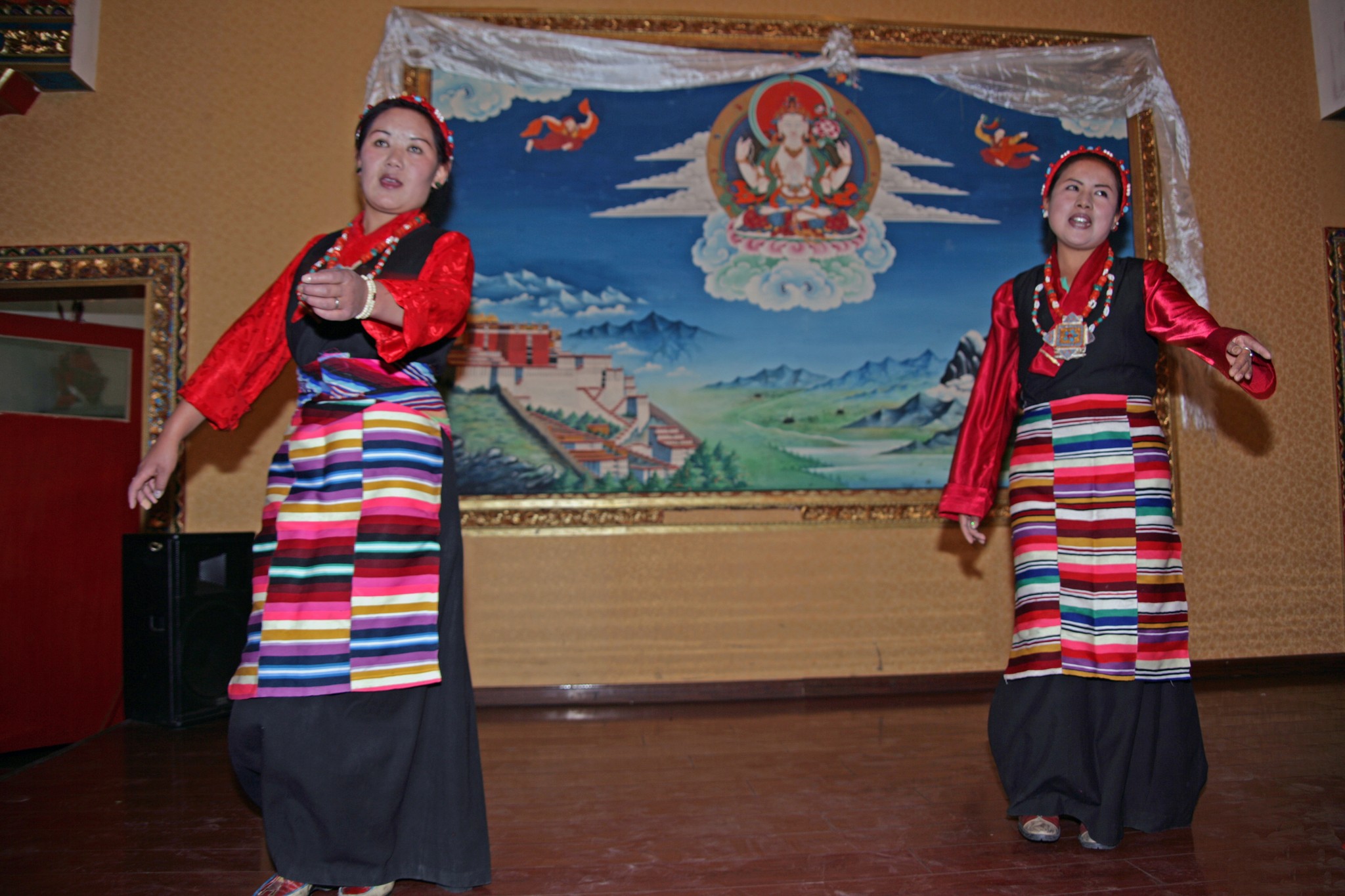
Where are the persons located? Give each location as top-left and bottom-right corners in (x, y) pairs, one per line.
(129, 92), (492, 896)
(937, 146), (1278, 851)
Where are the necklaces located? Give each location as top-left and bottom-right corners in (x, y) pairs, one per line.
(1031, 248), (1114, 345)
(296, 211), (425, 299)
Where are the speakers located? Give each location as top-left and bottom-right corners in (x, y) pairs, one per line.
(123, 532), (259, 725)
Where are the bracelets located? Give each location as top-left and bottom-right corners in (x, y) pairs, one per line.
(354, 274), (376, 319)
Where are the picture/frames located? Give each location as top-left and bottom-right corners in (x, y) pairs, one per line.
(401, 7), (1182, 529)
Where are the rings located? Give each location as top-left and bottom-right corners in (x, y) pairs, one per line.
(1243, 346), (1254, 358)
(334, 296), (339, 310)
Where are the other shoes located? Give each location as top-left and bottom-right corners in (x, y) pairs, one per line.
(252, 875), (334, 896)
(339, 880), (395, 896)
(1080, 821), (1118, 850)
(1018, 815), (1060, 840)
